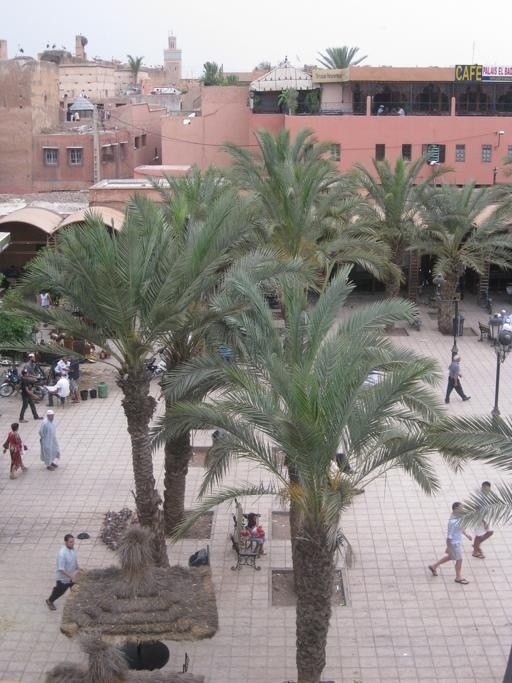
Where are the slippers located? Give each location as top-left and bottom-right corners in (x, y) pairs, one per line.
(428, 565), (439, 576)
(455, 577), (469, 584)
(471, 544), (485, 558)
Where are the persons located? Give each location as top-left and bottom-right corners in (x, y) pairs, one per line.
(329, 453), (365, 495)
(70, 110), (81, 122)
(46, 534), (82, 611)
(444, 354), (471, 404)
(35, 290), (52, 311)
(242, 512), (267, 558)
(2, 352), (80, 480)
(377, 104), (405, 116)
(488, 310), (512, 326)
(428, 481), (500, 584)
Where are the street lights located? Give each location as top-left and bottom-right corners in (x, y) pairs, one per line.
(484, 307), (512, 427)
(432, 272), (464, 361)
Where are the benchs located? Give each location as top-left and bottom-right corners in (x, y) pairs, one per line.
(230, 498), (262, 571)
(478, 321), (490, 343)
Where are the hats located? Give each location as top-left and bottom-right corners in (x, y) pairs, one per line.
(47, 410), (55, 415)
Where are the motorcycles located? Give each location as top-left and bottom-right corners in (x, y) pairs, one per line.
(408, 313), (423, 331)
(0, 356), (49, 403)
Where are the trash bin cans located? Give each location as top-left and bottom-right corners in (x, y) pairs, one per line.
(453, 314), (464, 336)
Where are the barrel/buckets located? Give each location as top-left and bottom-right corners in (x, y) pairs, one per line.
(79, 390), (88, 400)
(98, 384), (107, 398)
(89, 390), (97, 398)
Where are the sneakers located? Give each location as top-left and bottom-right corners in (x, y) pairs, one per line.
(46, 599), (57, 610)
(47, 463), (58, 471)
